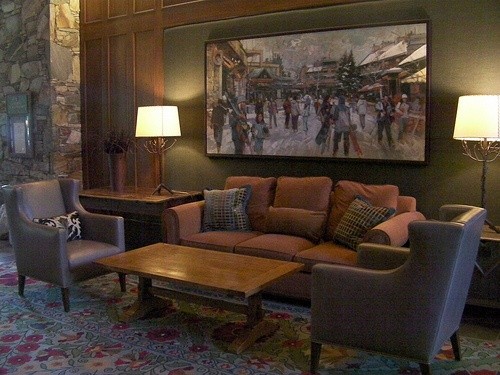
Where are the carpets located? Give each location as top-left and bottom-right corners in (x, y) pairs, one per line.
(0, 251), (500, 374)
(134, 105), (181, 195)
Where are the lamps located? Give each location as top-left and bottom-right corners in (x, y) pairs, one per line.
(453, 94), (500, 235)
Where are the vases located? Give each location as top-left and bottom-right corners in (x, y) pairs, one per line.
(110, 154), (128, 190)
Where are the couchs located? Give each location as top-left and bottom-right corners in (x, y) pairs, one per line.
(162, 193), (427, 303)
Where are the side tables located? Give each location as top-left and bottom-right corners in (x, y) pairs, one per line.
(79, 189), (201, 249)
(465, 225), (500, 311)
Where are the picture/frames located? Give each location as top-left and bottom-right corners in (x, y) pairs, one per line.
(205, 18), (433, 158)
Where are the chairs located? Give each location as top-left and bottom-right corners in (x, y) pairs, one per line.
(308, 205), (486, 375)
(0, 178), (127, 314)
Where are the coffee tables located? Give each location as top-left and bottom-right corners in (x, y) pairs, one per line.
(92, 246), (286, 354)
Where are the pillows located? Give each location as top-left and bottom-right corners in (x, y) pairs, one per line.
(273, 176), (333, 211)
(34, 211), (84, 241)
(204, 185), (255, 232)
(330, 195), (397, 250)
(264, 205), (330, 243)
(223, 175), (276, 233)
(326, 180), (398, 239)
